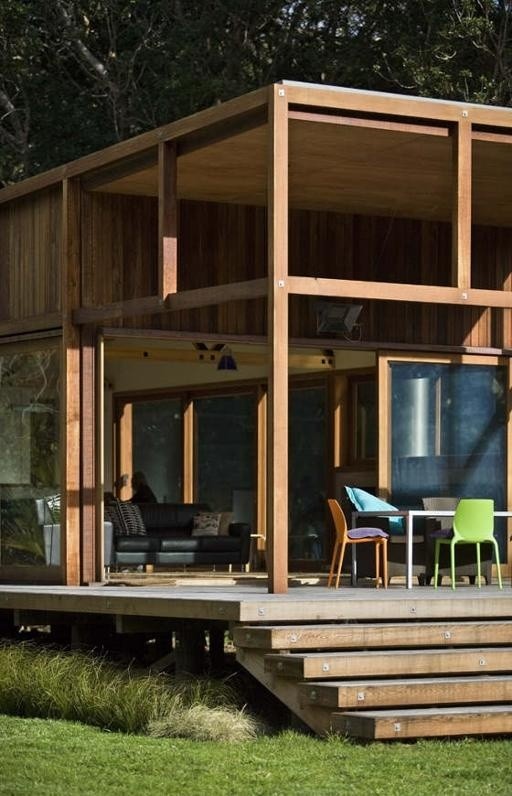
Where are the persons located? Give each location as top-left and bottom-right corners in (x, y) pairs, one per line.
(123, 471), (158, 504)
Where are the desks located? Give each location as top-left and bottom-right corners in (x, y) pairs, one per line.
(228, 533), (266, 573)
(352, 510), (512, 586)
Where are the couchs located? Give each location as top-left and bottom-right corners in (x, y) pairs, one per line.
(102, 502), (250, 580)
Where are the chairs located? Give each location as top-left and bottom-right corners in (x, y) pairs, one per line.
(422, 497), (493, 585)
(35, 497), (113, 574)
(357, 507), (426, 585)
(327, 498), (390, 591)
(434, 499), (502, 590)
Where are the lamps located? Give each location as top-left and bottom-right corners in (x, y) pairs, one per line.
(216, 347), (238, 377)
(317, 302), (369, 343)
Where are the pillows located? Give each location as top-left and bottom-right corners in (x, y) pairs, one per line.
(344, 486), (405, 535)
(346, 527), (390, 539)
(191, 513), (222, 536)
(104, 503), (147, 537)
(429, 525), (453, 539)
(43, 493), (61, 524)
(199, 511), (231, 537)
